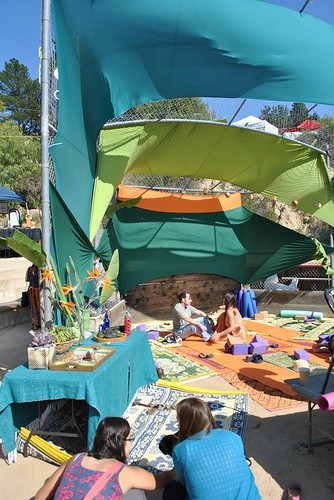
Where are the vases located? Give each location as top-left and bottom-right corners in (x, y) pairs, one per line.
(53, 339), (77, 354)
(68, 321), (84, 345)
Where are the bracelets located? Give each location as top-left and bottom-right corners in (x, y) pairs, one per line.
(209, 318), (211, 320)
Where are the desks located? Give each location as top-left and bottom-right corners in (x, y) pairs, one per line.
(89, 299), (126, 331)
(283, 373), (334, 454)
(0, 330), (159, 464)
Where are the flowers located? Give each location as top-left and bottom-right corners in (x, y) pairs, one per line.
(46, 325), (75, 342)
(0, 229), (119, 327)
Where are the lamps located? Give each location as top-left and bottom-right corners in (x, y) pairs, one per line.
(203, 190), (322, 209)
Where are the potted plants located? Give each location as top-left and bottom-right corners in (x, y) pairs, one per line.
(27, 329), (57, 370)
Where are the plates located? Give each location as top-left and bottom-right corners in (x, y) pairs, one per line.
(94, 332), (128, 341)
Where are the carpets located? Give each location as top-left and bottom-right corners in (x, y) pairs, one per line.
(17, 314), (334, 483)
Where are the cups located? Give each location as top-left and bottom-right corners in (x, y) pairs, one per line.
(299, 368), (309, 386)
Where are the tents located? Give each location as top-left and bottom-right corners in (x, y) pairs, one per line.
(0, 186), (30, 228)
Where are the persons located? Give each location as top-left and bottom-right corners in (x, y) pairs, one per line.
(162, 398), (263, 500)
(34, 417), (177, 500)
(26, 264), (41, 330)
(209, 293), (244, 342)
(172, 293), (214, 342)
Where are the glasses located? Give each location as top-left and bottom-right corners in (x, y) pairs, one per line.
(124, 436), (137, 444)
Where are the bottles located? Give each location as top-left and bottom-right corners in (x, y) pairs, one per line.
(101, 308), (110, 331)
(124, 312), (132, 336)
